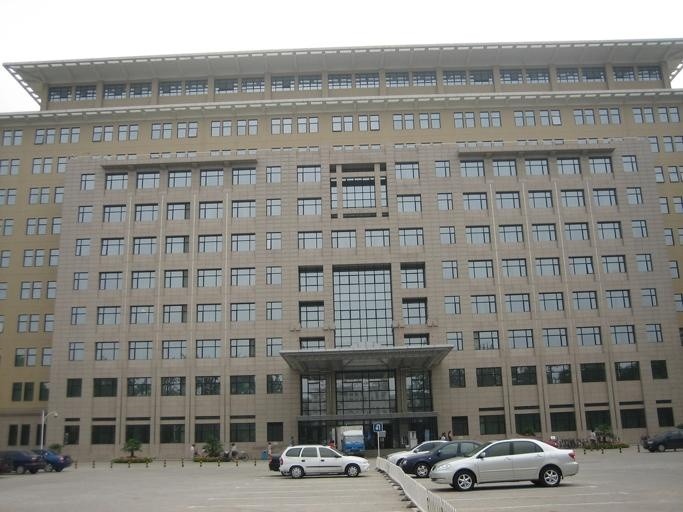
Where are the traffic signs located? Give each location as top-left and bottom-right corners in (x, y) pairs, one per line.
(372, 422), (383, 433)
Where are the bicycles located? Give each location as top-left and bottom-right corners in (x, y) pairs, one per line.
(224, 448), (249, 461)
(550, 439), (602, 451)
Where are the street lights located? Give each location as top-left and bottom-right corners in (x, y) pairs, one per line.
(40, 410), (58, 450)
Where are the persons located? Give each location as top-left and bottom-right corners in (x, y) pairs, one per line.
(328, 440), (334, 448)
(448, 431), (452, 441)
(591, 429), (597, 445)
(440, 433), (447, 440)
(191, 444), (197, 456)
(231, 443), (237, 457)
(268, 443), (272, 457)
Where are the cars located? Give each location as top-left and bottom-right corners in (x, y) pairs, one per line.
(31, 449), (71, 473)
(343, 442), (365, 457)
(385, 440), (448, 466)
(0, 449), (45, 475)
(268, 454), (280, 471)
(428, 439), (579, 492)
(643, 430), (682, 452)
(397, 440), (483, 479)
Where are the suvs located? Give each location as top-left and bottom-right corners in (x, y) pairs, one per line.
(278, 445), (369, 479)
